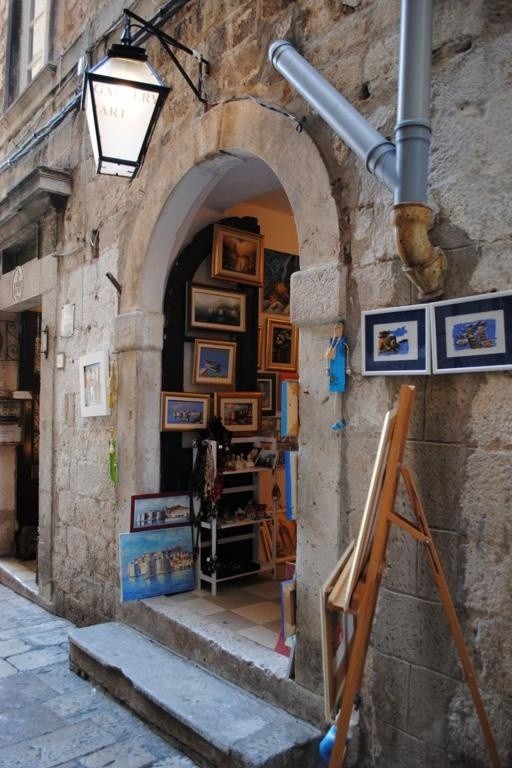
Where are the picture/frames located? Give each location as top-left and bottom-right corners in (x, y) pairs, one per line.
(213, 392), (263, 435)
(212, 225), (265, 287)
(192, 338), (236, 387)
(430, 288), (512, 375)
(264, 318), (297, 370)
(160, 391), (211, 431)
(360, 304), (430, 376)
(257, 325), (264, 368)
(184, 280), (250, 343)
(79, 351), (110, 418)
(257, 372), (278, 416)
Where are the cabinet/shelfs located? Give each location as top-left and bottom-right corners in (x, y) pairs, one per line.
(199, 468), (277, 596)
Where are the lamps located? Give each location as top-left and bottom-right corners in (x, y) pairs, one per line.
(79, 9), (209, 178)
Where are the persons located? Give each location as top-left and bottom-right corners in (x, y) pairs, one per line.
(223, 451), (274, 472)
(324, 323), (351, 431)
(275, 333), (289, 363)
(378, 334), (400, 355)
